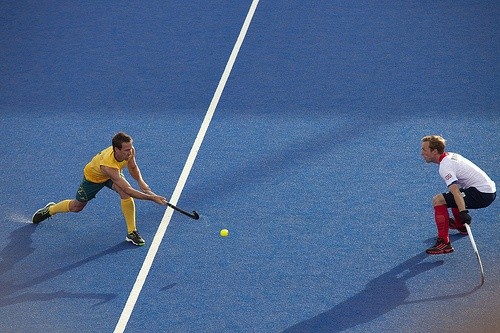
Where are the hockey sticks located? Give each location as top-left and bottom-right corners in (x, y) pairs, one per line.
(465, 223), (485, 286)
(146, 190), (200, 220)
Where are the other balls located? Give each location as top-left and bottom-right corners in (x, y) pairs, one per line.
(220, 229), (229, 237)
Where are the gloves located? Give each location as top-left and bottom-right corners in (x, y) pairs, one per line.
(458, 210), (471, 225)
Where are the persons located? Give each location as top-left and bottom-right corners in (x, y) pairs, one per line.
(421, 136), (496, 254)
(32, 131), (166, 245)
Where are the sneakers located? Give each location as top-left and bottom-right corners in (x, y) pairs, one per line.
(426, 237), (455, 254)
(32, 202), (56, 224)
(125, 229), (145, 245)
(449, 218), (468, 235)
